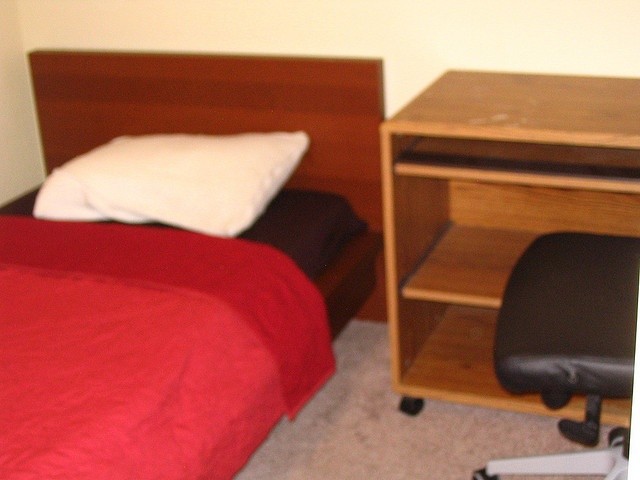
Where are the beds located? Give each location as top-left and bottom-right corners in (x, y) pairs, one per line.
(0, 49), (386, 480)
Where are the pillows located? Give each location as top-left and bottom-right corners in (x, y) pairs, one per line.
(30, 129), (312, 239)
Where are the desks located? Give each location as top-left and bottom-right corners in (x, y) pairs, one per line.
(378, 68), (640, 434)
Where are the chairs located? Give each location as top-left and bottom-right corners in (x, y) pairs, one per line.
(471, 229), (640, 480)
